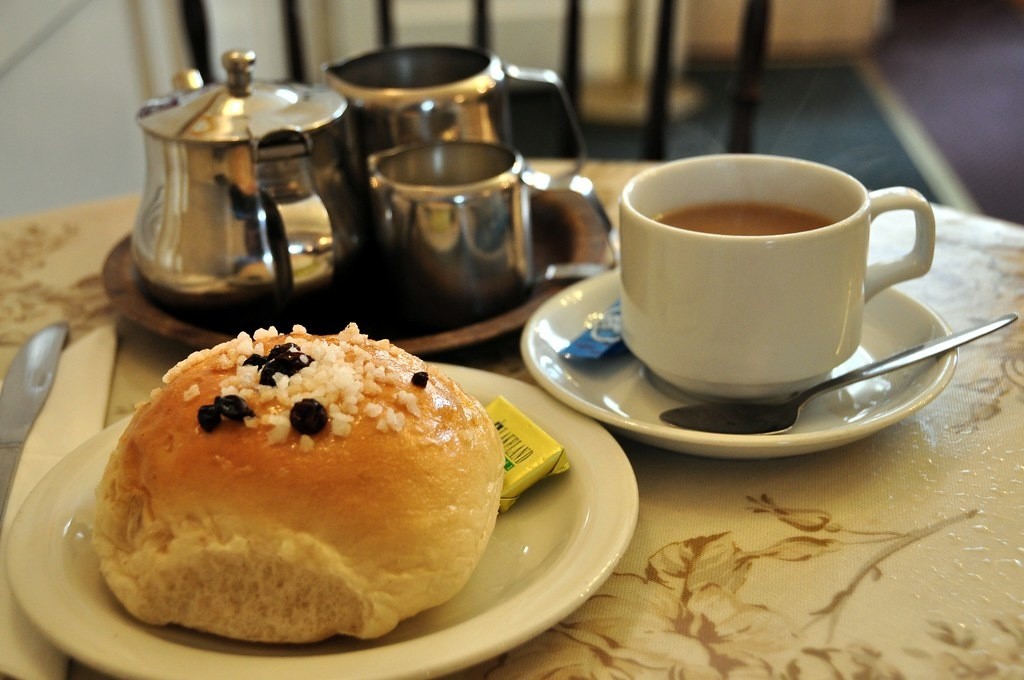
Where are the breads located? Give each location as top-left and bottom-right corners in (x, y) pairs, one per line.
(91, 324), (510, 643)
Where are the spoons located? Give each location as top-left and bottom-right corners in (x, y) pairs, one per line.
(659, 312), (1019, 436)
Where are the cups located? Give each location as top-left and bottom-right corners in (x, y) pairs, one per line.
(620, 152), (936, 399)
(324, 44), (586, 177)
(367, 139), (617, 304)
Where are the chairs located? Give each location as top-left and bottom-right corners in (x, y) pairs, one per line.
(179, 1), (771, 163)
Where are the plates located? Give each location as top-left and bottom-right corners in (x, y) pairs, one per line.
(522, 269), (958, 459)
(7, 362), (640, 680)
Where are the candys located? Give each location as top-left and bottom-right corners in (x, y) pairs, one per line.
(485, 394), (572, 513)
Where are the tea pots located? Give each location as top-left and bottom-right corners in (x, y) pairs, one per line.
(131, 48), (361, 307)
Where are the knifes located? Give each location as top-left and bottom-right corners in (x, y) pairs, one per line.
(0, 322), (69, 512)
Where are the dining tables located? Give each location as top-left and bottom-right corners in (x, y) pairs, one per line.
(0, 159), (1024, 679)
